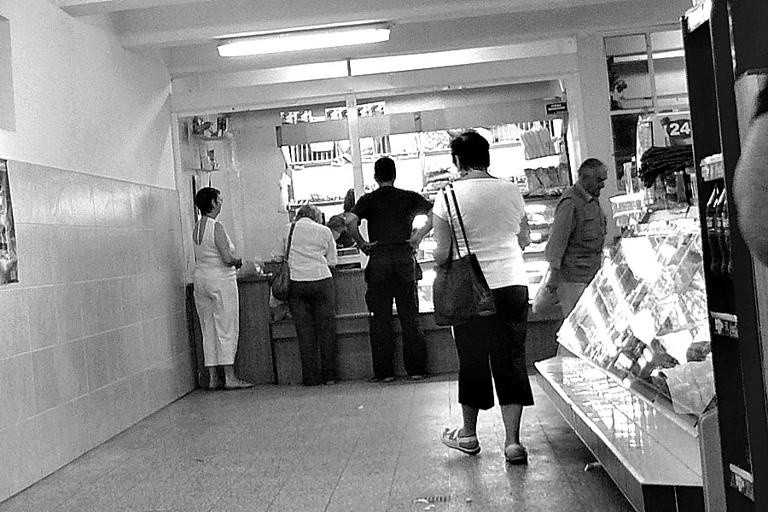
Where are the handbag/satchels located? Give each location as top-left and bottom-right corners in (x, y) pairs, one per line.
(270, 219), (295, 300)
(433, 182), (498, 326)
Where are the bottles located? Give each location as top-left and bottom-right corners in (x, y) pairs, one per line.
(706, 184), (734, 277)
(656, 167), (699, 207)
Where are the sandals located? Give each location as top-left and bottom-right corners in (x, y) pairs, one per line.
(504, 443), (529, 465)
(439, 426), (481, 455)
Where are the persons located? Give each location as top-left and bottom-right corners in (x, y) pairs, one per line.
(268, 204), (338, 387)
(544, 158), (608, 357)
(191, 187), (256, 391)
(732, 75), (768, 267)
(326, 188), (355, 249)
(345, 156), (433, 384)
(432, 130), (535, 466)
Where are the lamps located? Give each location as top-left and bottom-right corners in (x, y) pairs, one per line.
(216, 20), (393, 56)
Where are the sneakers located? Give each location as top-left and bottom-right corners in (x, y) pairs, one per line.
(368, 375), (394, 383)
(410, 372), (426, 380)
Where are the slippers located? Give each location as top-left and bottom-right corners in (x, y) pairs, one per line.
(225, 379), (254, 389)
(207, 382), (223, 391)
